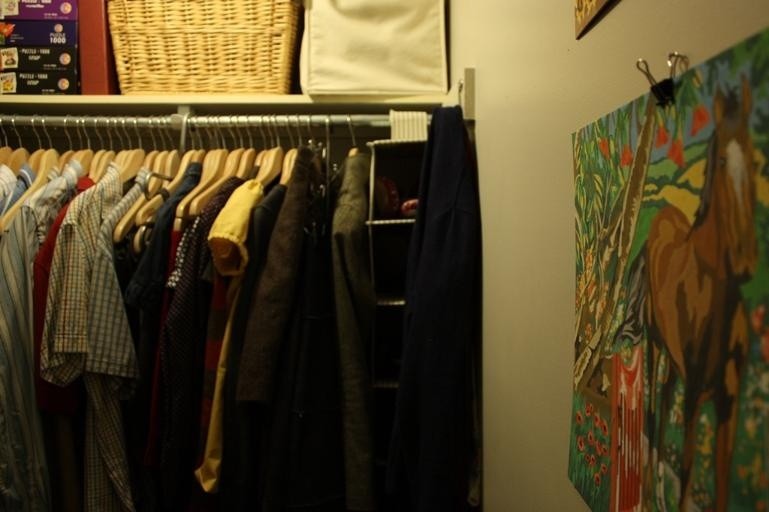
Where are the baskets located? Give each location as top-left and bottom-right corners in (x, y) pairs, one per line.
(105, 0), (297, 98)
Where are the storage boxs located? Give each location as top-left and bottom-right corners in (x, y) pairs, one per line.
(298, 0), (451, 99)
